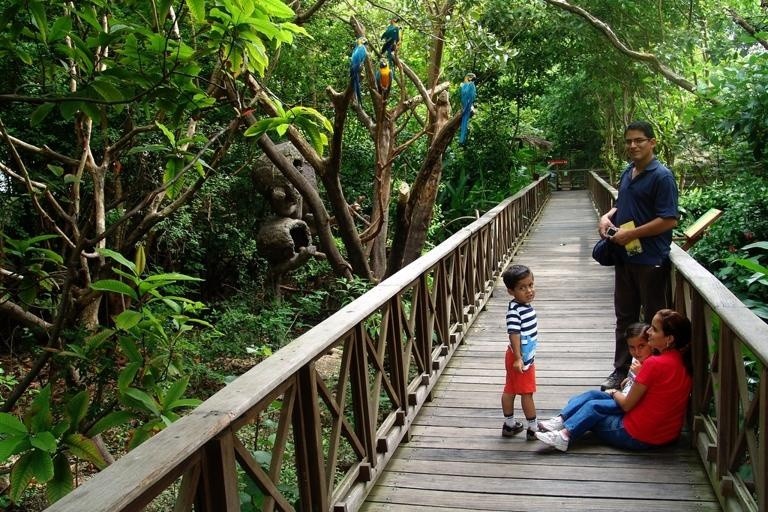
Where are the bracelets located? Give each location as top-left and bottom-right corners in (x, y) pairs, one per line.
(609, 389), (617, 398)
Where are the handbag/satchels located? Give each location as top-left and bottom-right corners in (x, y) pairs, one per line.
(592, 222), (622, 266)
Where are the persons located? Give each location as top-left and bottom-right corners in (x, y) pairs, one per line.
(502, 266), (550, 440)
(568, 308), (694, 453)
(598, 122), (678, 390)
(534, 323), (654, 452)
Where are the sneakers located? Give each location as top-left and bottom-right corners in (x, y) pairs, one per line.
(526, 427), (544, 441)
(502, 421), (523, 436)
(538, 417), (564, 432)
(534, 430), (569, 452)
(601, 372), (627, 391)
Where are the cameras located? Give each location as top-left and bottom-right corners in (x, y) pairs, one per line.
(606, 226), (618, 238)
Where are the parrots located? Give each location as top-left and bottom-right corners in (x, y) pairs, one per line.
(381, 16), (400, 79)
(459, 73), (478, 146)
(350, 36), (368, 110)
(375, 58), (393, 100)
